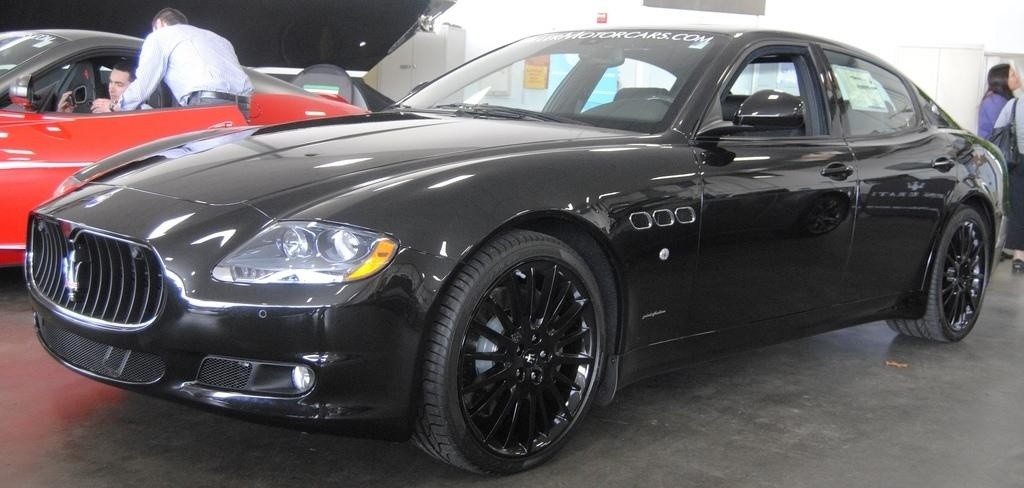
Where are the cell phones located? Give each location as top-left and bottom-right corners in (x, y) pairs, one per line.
(66, 95), (74, 106)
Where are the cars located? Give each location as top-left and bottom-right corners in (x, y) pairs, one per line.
(0, 29), (372, 268)
(24, 29), (1010, 474)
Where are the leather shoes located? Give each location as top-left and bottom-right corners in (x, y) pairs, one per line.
(1013, 258), (1023, 273)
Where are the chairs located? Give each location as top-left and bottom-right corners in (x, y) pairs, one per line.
(587, 88), (806, 137)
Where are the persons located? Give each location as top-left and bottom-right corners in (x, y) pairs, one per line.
(993, 97), (1024, 272)
(976, 64), (1020, 139)
(57, 58), (153, 112)
(90, 6), (252, 125)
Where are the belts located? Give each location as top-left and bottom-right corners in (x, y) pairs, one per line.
(187, 91), (253, 105)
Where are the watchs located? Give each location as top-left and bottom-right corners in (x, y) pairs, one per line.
(109, 99), (117, 112)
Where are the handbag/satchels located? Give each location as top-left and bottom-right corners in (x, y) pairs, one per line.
(987, 122), (1022, 167)
